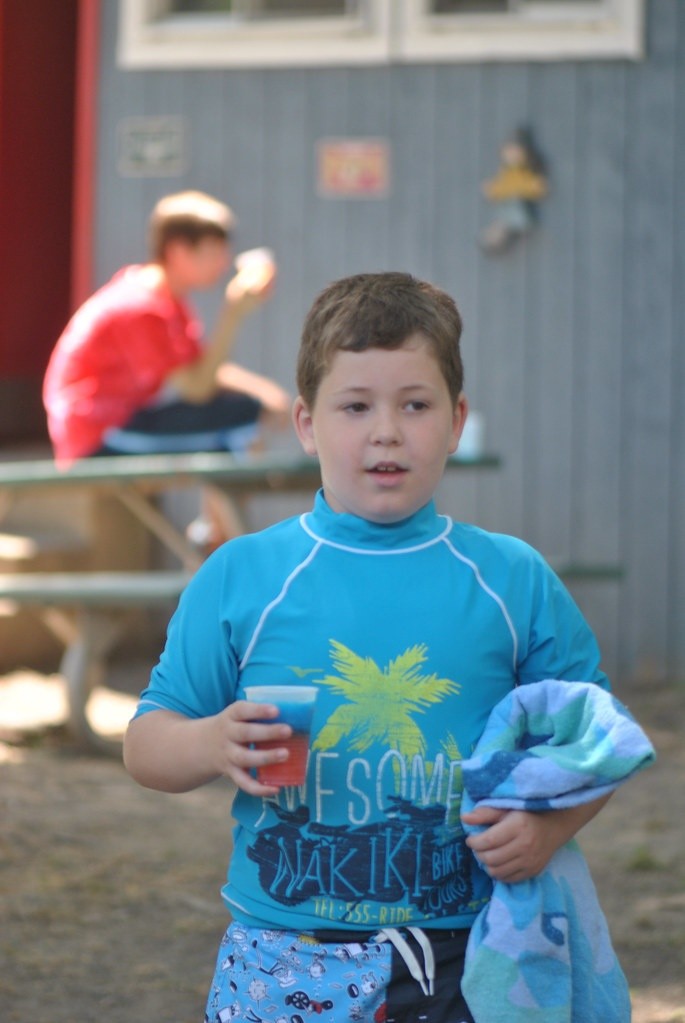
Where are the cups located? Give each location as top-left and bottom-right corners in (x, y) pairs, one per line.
(244, 686), (317, 786)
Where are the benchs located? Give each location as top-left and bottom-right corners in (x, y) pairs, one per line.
(0, 560), (626, 755)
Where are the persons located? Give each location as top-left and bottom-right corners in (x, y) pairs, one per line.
(43, 189), (290, 554)
(123, 269), (618, 1022)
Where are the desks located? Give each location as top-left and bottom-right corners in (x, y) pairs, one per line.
(0, 450), (504, 569)
